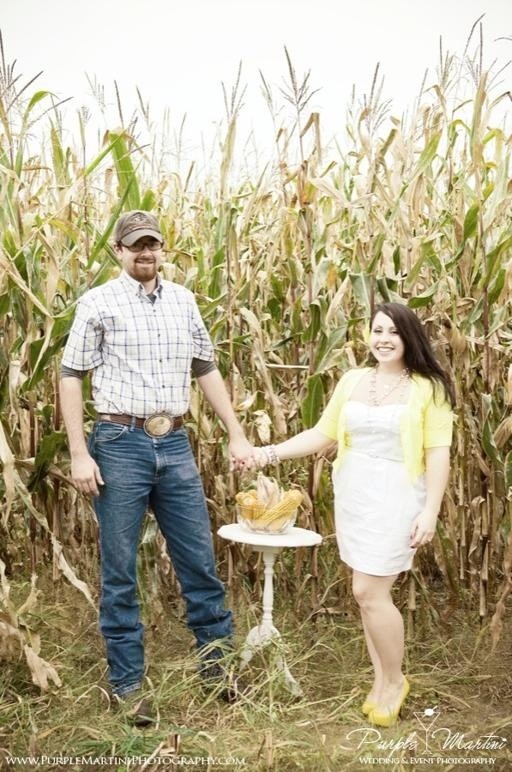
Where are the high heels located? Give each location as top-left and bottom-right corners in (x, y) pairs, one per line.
(369, 675), (411, 726)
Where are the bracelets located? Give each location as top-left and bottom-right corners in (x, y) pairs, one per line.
(263, 441), (282, 466)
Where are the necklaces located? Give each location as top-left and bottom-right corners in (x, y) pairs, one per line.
(366, 362), (412, 408)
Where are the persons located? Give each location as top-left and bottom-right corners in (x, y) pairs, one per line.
(56, 210), (251, 729)
(230, 301), (458, 729)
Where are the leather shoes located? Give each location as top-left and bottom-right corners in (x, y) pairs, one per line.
(362, 688), (374, 715)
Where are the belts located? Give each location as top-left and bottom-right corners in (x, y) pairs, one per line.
(97, 411), (185, 439)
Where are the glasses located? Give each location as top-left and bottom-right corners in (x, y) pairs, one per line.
(119, 240), (163, 252)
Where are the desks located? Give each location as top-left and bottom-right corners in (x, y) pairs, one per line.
(216, 524), (322, 704)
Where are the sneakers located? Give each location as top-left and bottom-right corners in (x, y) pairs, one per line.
(115, 692), (152, 726)
(201, 648), (232, 694)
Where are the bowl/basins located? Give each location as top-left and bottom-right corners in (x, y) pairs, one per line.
(234, 503), (300, 536)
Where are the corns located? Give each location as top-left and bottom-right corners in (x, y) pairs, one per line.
(0, 192), (512, 531)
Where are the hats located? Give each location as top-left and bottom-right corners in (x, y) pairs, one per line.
(113, 210), (163, 247)
